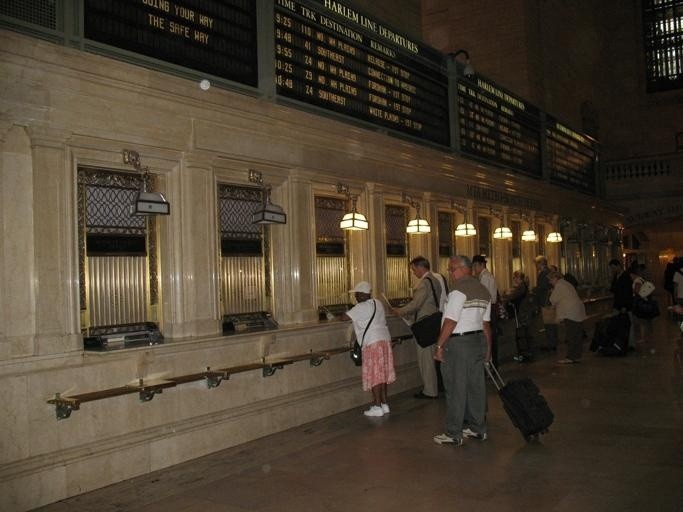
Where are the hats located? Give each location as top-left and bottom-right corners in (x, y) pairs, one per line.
(470, 256), (486, 263)
(348, 282), (373, 295)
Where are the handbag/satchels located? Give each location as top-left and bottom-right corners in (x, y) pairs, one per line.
(505, 282), (527, 318)
(541, 290), (559, 325)
(635, 279), (653, 300)
(410, 277), (442, 347)
(350, 299), (377, 366)
(495, 289), (508, 323)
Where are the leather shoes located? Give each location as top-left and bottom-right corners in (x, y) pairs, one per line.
(414, 391), (437, 399)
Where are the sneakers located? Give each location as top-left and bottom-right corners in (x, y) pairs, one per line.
(461, 428), (483, 441)
(364, 406), (383, 417)
(557, 356), (573, 364)
(380, 402), (389, 413)
(432, 432), (463, 446)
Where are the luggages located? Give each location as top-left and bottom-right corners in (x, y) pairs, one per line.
(484, 361), (554, 439)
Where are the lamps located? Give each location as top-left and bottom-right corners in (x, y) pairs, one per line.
(673, 132), (683, 153)
(546, 214), (563, 243)
(123, 148), (170, 217)
(402, 194), (431, 236)
(339, 199), (369, 231)
(451, 200), (477, 238)
(521, 212), (538, 241)
(448, 50), (475, 76)
(247, 170), (287, 226)
(490, 206), (513, 240)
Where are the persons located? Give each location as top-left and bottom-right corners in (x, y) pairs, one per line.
(629, 270), (648, 343)
(435, 255), (493, 446)
(506, 271), (533, 362)
(345, 281), (391, 418)
(672, 266), (682, 325)
(608, 260), (638, 348)
(534, 256), (552, 333)
(472, 255), (499, 364)
(430, 270), (448, 312)
(393, 257), (443, 400)
(547, 271), (586, 364)
